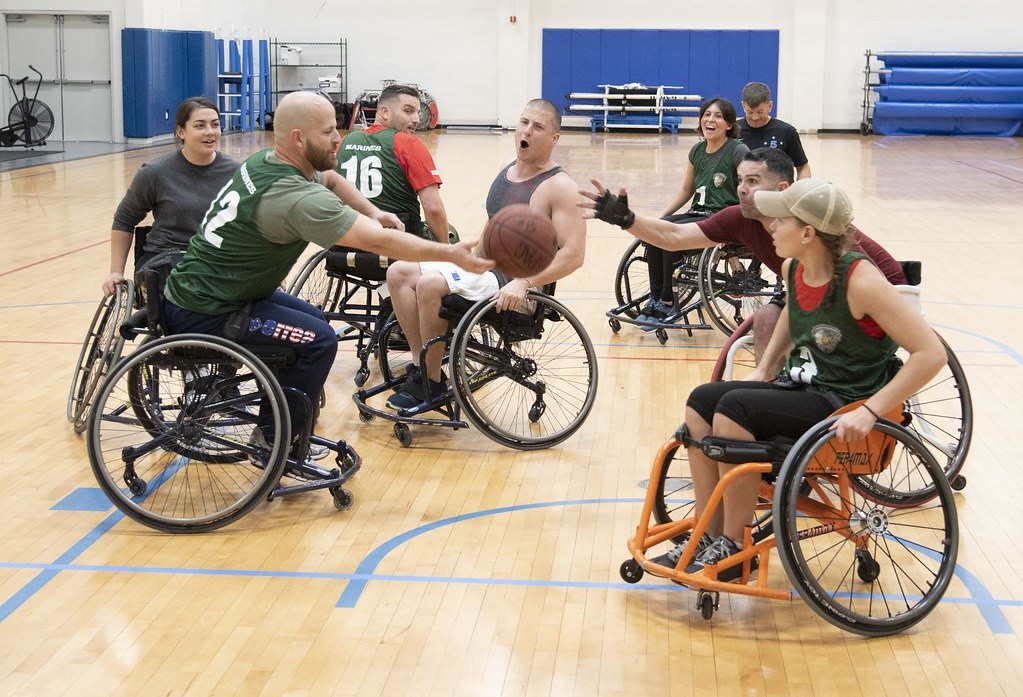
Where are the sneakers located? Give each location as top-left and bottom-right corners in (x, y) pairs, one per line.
(676, 535), (760, 593)
(387, 370), (447, 411)
(646, 533), (717, 579)
(641, 299), (684, 333)
(634, 296), (657, 329)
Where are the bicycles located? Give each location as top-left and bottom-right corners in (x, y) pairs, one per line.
(0, 65), (55, 148)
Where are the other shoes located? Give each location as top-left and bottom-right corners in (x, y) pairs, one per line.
(184, 370), (212, 407)
(216, 376), (247, 419)
(248, 430), (325, 484)
(306, 443), (329, 460)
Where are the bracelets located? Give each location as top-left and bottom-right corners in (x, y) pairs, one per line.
(861, 403), (880, 421)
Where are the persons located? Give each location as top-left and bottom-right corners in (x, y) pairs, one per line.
(386, 99), (587, 410)
(636, 99), (751, 332)
(728, 81), (812, 298)
(331, 84), (451, 352)
(575, 148), (907, 381)
(646, 178), (948, 591)
(102, 98), (245, 418)
(163, 91), (495, 466)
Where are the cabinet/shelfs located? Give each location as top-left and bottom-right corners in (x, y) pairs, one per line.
(268, 37), (348, 122)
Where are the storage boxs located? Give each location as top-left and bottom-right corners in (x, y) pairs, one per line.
(319, 77), (342, 92)
(280, 46), (300, 65)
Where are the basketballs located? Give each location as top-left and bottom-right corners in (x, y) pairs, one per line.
(483, 203), (558, 278)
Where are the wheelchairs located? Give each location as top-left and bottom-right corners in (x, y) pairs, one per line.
(87, 245), (363, 534)
(619, 256), (975, 636)
(605, 185), (787, 345)
(66, 225), (327, 433)
(353, 228), (598, 453)
(283, 210), (492, 401)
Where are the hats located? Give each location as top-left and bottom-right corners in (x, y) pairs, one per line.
(753, 179), (854, 237)
